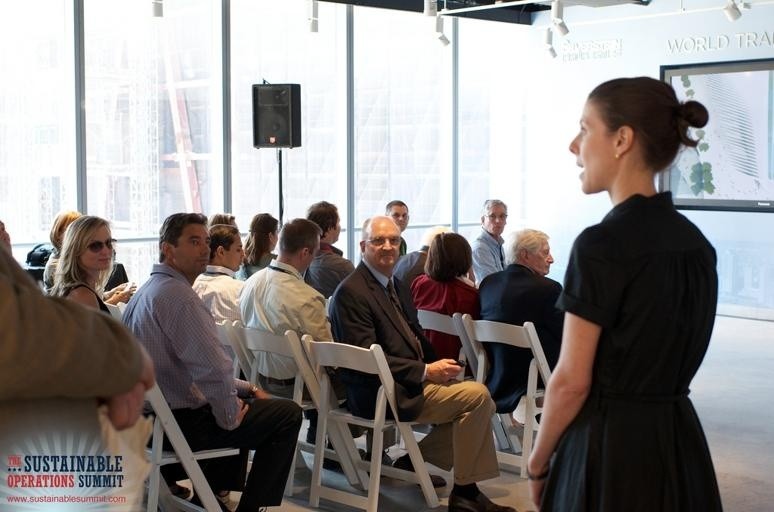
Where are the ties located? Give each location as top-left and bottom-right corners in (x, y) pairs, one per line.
(387, 279), (421, 358)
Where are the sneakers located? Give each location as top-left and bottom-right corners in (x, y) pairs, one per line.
(225, 500), (268, 512)
(306, 428), (391, 478)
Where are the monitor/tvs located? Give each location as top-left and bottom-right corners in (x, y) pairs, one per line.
(655, 56), (774, 216)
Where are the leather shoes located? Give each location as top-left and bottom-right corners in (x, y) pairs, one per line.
(394, 457), (447, 489)
(448, 493), (515, 512)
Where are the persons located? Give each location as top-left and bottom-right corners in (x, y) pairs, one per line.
(0, 193), (563, 511)
(526, 76), (723, 512)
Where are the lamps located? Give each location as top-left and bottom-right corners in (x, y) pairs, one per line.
(423, 0), (438, 18)
(434, 16), (451, 48)
(150, 0), (164, 18)
(721, 1), (742, 24)
(305, 1), (319, 32)
(543, 28), (558, 59)
(551, 0), (570, 38)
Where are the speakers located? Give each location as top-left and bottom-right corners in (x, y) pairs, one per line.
(251, 82), (304, 151)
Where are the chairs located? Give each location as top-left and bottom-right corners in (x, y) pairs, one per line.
(142, 296), (555, 512)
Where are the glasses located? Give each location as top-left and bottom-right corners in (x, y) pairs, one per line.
(87, 239), (117, 253)
(367, 236), (402, 247)
(486, 214), (508, 219)
